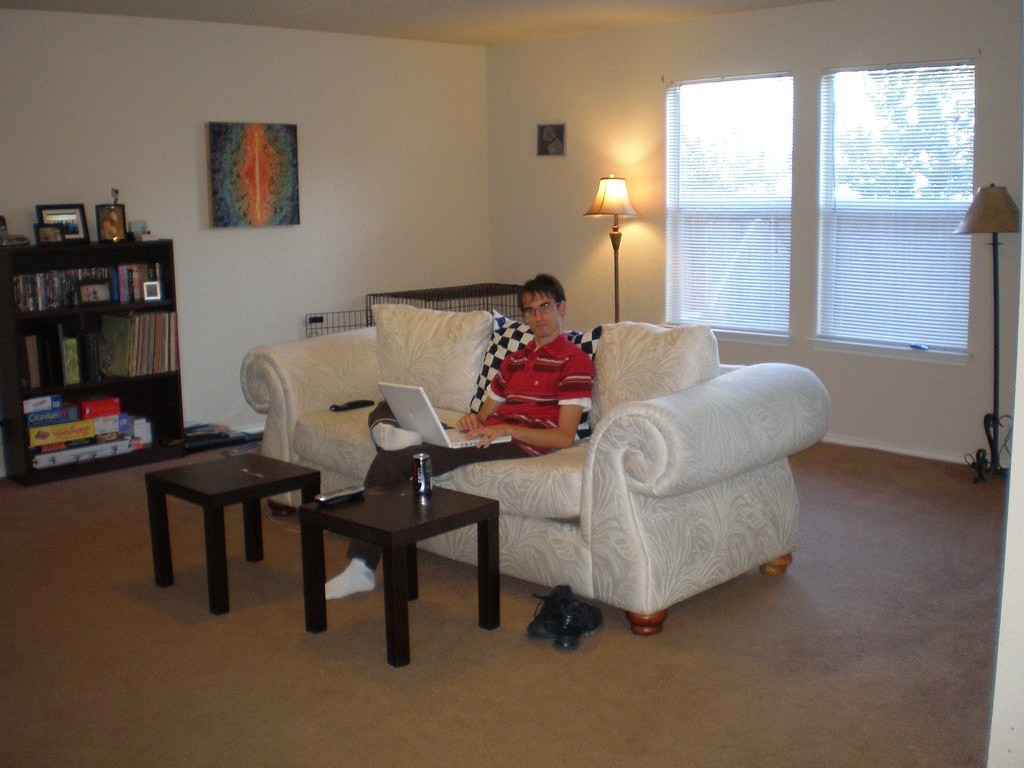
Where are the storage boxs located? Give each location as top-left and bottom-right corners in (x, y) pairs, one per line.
(23, 394), (62, 414)
(26, 402), (78, 427)
(65, 394), (120, 420)
(93, 412), (128, 436)
(131, 421), (152, 442)
(26, 418), (95, 446)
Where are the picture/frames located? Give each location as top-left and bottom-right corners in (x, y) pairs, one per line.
(35, 222), (66, 246)
(35, 203), (90, 246)
(95, 204), (126, 243)
(534, 122), (568, 156)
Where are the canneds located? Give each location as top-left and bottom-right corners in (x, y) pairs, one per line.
(412, 453), (433, 493)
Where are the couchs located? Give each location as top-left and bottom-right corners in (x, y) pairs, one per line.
(242, 328), (830, 637)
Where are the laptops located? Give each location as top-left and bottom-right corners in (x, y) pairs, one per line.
(377, 382), (511, 448)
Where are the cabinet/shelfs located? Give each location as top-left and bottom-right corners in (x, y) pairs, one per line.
(0, 240), (186, 486)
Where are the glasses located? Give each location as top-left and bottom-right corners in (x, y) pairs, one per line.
(522, 300), (562, 319)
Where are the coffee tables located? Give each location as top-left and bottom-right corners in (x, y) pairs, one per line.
(298, 479), (500, 667)
(146, 453), (319, 615)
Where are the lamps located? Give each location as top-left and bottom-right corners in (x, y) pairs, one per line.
(583, 173), (640, 324)
(953, 184), (1022, 484)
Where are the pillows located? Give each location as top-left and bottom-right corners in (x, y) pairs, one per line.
(468, 311), (603, 441)
(590, 321), (720, 435)
(368, 304), (493, 414)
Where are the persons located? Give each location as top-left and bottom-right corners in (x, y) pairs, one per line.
(325, 274), (596, 622)
(98, 210), (123, 241)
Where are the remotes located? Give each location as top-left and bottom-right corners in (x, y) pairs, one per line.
(313, 485), (364, 505)
(330, 400), (374, 412)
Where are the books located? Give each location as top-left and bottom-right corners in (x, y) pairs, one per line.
(14, 262), (178, 388)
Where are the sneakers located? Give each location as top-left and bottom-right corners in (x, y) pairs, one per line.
(553, 602), (603, 650)
(527, 585), (572, 639)
